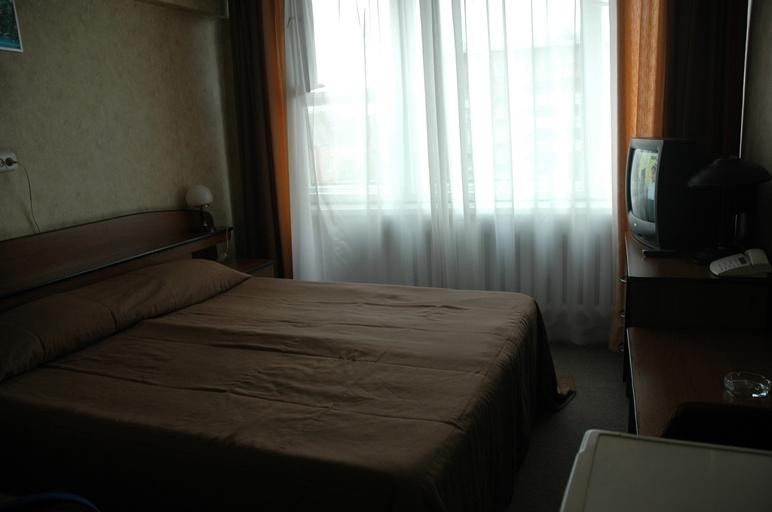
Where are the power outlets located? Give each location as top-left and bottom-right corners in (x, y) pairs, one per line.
(0, 152), (18, 172)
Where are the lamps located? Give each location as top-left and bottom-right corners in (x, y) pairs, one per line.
(186, 184), (214, 238)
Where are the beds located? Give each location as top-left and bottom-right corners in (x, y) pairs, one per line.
(0, 206), (575, 512)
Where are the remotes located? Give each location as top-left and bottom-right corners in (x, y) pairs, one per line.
(642, 250), (679, 258)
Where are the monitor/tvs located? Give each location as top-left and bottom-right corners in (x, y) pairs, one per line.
(625, 136), (715, 250)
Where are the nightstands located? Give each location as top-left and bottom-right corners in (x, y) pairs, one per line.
(216, 251), (275, 277)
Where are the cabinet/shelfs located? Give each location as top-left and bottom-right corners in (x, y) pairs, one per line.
(618, 231), (772, 380)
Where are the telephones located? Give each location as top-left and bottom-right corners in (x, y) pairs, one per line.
(708, 248), (771, 279)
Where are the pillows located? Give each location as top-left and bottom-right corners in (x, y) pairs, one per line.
(0, 291), (116, 385)
(66, 258), (253, 331)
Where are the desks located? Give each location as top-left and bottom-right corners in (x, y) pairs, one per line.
(624, 325), (772, 450)
(559, 429), (772, 512)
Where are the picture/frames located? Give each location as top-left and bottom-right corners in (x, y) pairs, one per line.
(0, 0), (23, 53)
